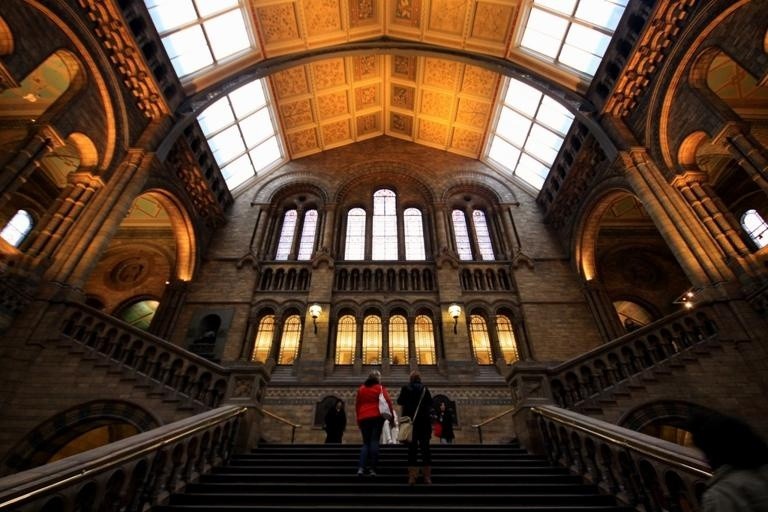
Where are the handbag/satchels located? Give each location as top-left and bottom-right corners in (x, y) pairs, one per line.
(379, 393), (393, 420)
(398, 416), (412, 443)
(434, 423), (442, 437)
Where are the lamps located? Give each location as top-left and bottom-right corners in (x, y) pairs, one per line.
(448, 303), (462, 335)
(309, 302), (322, 335)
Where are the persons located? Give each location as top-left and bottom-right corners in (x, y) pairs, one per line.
(436, 402), (454, 444)
(324, 399), (346, 443)
(356, 370), (434, 484)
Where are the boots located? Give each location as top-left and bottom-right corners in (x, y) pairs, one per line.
(408, 467), (431, 485)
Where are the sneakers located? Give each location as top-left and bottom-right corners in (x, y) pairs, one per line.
(358, 467), (376, 476)
